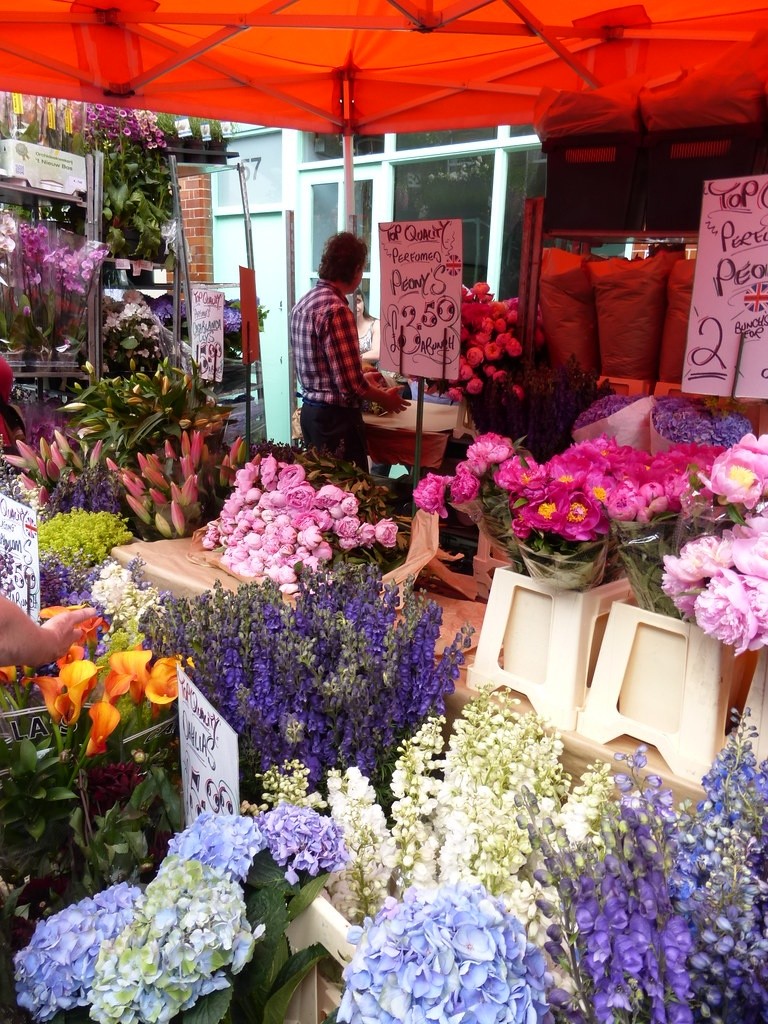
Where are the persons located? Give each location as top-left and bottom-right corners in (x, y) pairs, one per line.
(0, 594), (96, 670)
(347, 287), (381, 368)
(287, 231), (411, 480)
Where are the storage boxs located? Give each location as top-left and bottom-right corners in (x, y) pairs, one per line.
(468, 565), (638, 732)
(576, 597), (756, 786)
(636, 85), (766, 230)
(534, 94), (647, 228)
(279, 879), (370, 1024)
(1, 138), (88, 195)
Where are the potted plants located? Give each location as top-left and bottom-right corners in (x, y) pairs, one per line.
(181, 115), (207, 162)
(155, 112), (188, 163)
(206, 119), (234, 165)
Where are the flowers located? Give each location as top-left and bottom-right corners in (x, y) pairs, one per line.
(0, 92), (768, 1024)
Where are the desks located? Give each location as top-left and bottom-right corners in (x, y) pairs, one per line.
(112, 539), (712, 813)
(292, 399), (474, 469)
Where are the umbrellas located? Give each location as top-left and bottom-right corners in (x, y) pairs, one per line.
(1, 0), (768, 235)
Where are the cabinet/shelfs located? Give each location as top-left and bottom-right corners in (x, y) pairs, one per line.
(0, 147), (267, 450)
(517, 196), (768, 377)
